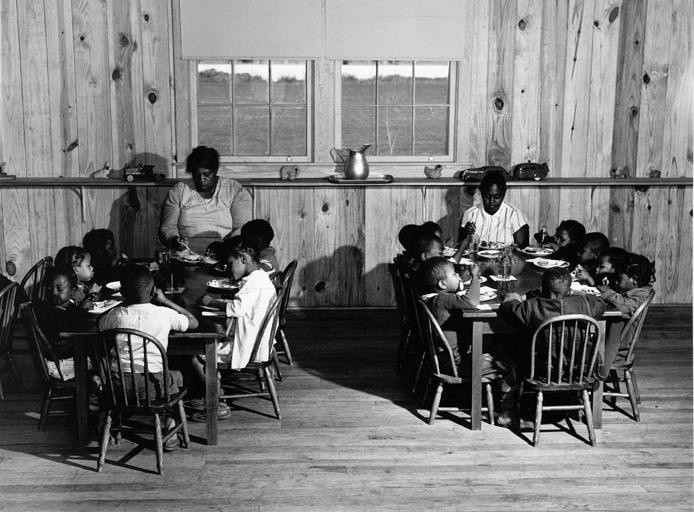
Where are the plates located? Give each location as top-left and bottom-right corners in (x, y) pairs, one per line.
(523, 244), (570, 270)
(206, 278), (238, 291)
(443, 247), (512, 304)
(86, 279), (123, 315)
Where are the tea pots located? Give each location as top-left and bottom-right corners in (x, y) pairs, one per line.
(337, 143), (375, 180)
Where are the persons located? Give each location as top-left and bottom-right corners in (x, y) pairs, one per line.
(36, 219), (282, 424)
(158, 146), (253, 253)
(87, 263), (200, 452)
(396, 219), (654, 429)
(457, 173), (530, 250)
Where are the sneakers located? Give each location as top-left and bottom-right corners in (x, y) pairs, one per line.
(163, 389), (231, 451)
